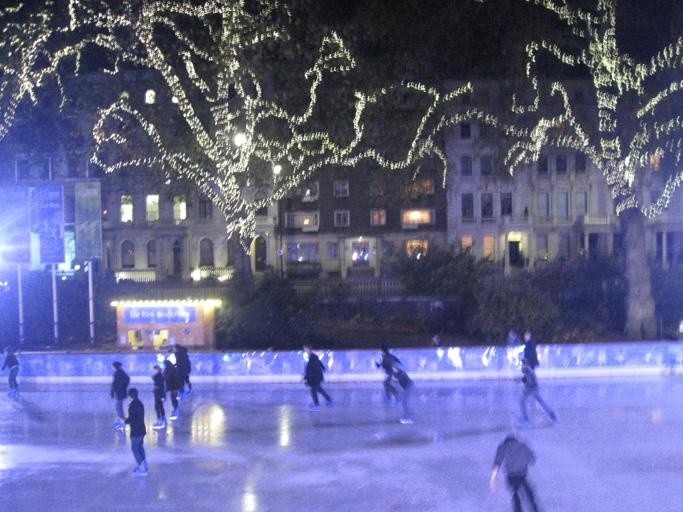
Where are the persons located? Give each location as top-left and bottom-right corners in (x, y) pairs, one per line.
(521, 330), (540, 384)
(152, 344), (192, 427)
(1, 346), (20, 397)
(120, 389), (147, 473)
(303, 344), (335, 412)
(519, 358), (557, 425)
(489, 436), (540, 512)
(392, 366), (415, 424)
(376, 344), (402, 402)
(111, 362), (129, 429)
